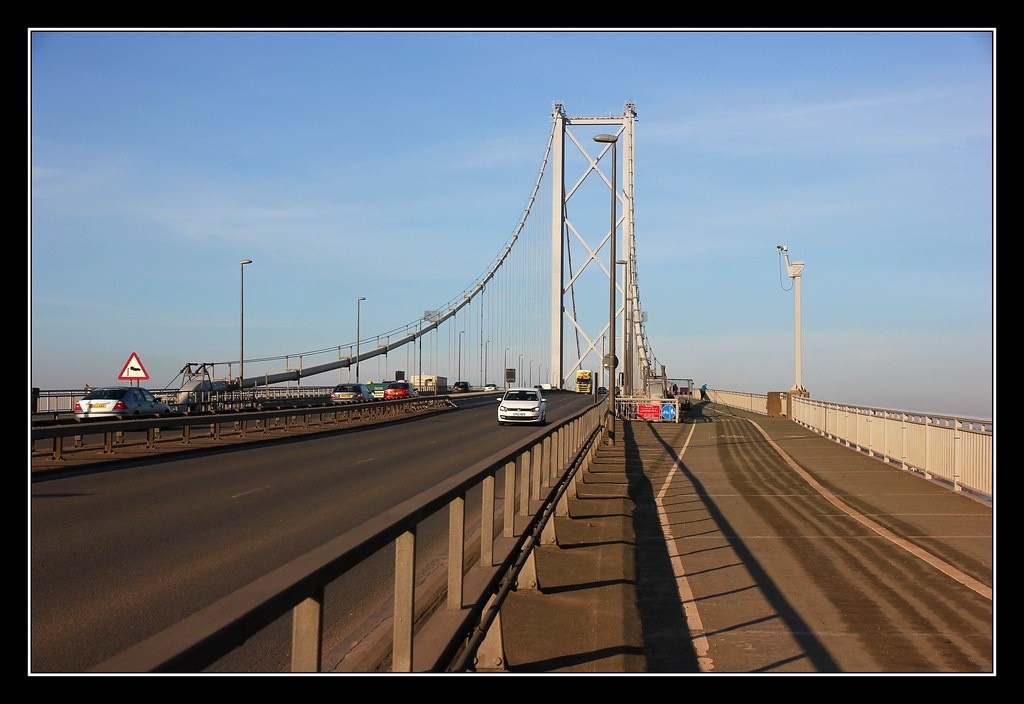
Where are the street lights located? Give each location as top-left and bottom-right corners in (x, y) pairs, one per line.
(539, 364), (542, 385)
(239, 259), (253, 404)
(593, 134), (634, 447)
(458, 330), (465, 382)
(356, 296), (366, 383)
(504, 348), (509, 388)
(485, 340), (490, 383)
(529, 359), (533, 388)
(419, 317), (427, 392)
(518, 354), (523, 388)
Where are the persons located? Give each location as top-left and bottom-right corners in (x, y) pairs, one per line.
(673, 383), (678, 395)
(700, 383), (708, 401)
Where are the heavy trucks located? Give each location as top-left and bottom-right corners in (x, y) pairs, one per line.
(575, 369), (592, 394)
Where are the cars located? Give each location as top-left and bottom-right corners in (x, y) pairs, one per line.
(453, 382), (473, 393)
(74, 386), (172, 417)
(484, 383), (499, 391)
(496, 388), (547, 426)
(330, 382), (416, 405)
(598, 387), (608, 395)
